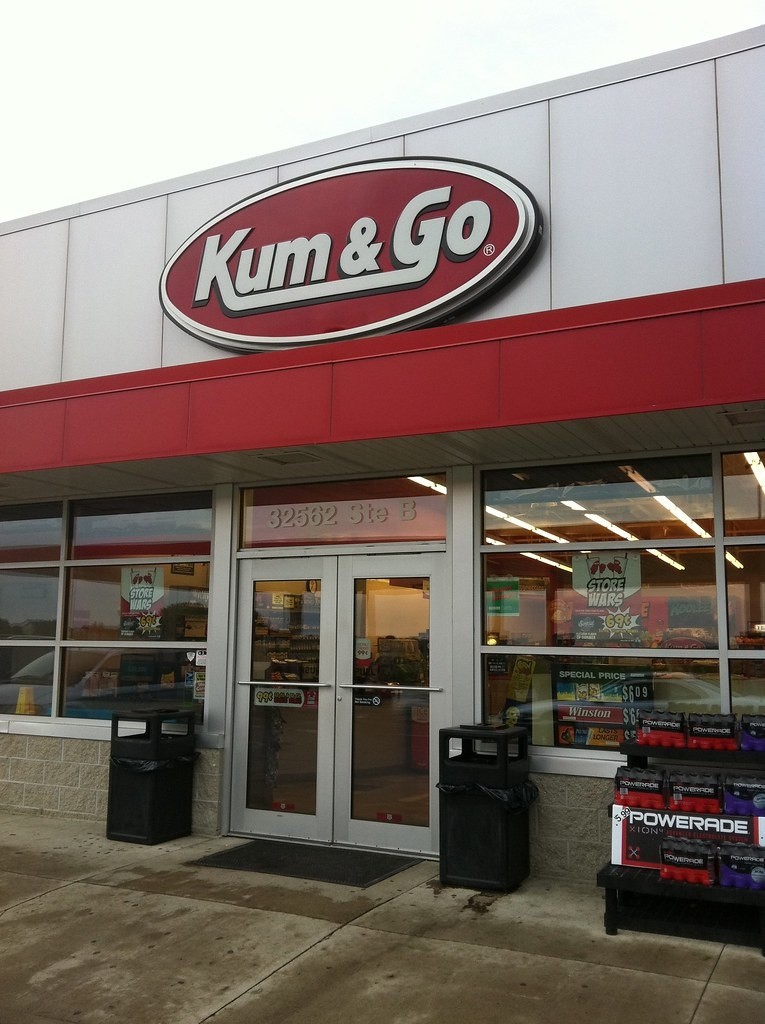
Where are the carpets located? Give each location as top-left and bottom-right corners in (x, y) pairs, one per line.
(185, 839), (424, 888)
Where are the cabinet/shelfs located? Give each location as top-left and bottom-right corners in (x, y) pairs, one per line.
(596, 738), (764, 958)
(255, 609), (320, 681)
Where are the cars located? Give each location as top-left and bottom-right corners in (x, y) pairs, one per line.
(490, 671), (765, 750)
(1, 647), (177, 721)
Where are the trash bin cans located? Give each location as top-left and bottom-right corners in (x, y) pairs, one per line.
(436, 722), (535, 895)
(105, 703), (198, 846)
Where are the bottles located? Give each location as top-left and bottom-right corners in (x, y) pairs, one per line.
(637, 709), (765, 750)
(614, 766), (765, 817)
(658, 834), (765, 889)
